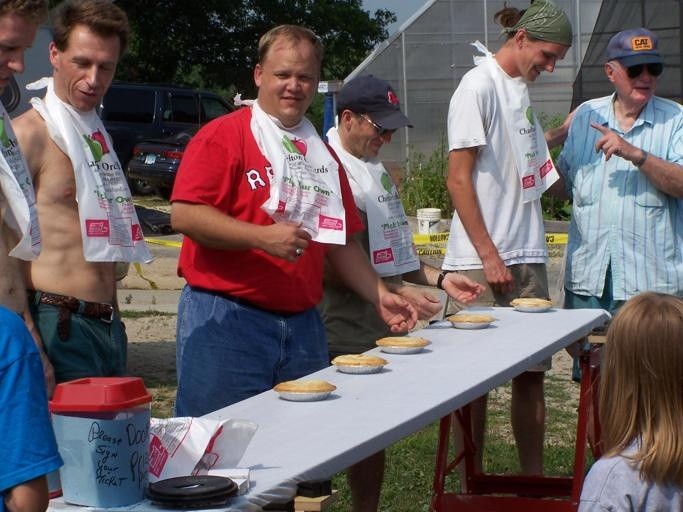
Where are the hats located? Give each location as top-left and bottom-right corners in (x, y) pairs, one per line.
(607, 28), (664, 69)
(336, 74), (414, 130)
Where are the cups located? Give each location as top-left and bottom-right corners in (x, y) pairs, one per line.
(417, 207), (443, 235)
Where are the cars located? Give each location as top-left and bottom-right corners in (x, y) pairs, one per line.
(128, 131), (194, 196)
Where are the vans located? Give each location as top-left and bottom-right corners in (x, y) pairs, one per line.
(95, 85), (246, 193)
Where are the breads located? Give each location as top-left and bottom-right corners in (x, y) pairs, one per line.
(511, 298), (553, 307)
(447, 314), (496, 323)
(273, 380), (337, 393)
(332, 354), (387, 366)
(376, 337), (431, 347)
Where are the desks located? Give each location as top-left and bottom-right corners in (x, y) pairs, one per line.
(49, 302), (611, 512)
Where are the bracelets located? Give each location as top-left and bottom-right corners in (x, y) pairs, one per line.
(632, 150), (648, 168)
(436, 270), (450, 290)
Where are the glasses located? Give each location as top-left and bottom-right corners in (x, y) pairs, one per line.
(356, 112), (397, 135)
(628, 63), (663, 79)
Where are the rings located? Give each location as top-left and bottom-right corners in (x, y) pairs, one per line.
(295, 248), (301, 256)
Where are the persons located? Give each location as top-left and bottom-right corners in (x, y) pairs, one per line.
(11, 0), (150, 421)
(580, 292), (682, 512)
(1, 0), (48, 315)
(543, 23), (682, 383)
(0, 303), (62, 512)
(315, 71), (486, 511)
(443, 1), (581, 504)
(170, 22), (418, 512)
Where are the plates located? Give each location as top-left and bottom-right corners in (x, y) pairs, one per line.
(509, 302), (556, 312)
(379, 337), (431, 354)
(274, 385), (338, 401)
(449, 317), (495, 328)
(332, 358), (387, 374)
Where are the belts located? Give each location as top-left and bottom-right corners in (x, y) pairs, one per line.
(40, 292), (119, 342)
